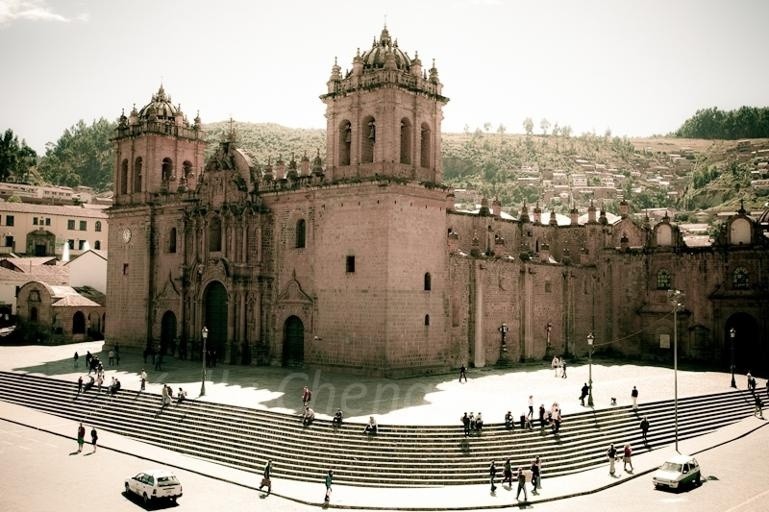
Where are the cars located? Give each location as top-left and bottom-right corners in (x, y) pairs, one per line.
(653, 456), (701, 488)
(124, 469), (183, 504)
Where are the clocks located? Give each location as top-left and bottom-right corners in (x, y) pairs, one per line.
(121, 228), (133, 243)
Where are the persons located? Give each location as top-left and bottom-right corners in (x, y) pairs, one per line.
(459, 364), (467, 382)
(302, 385), (344, 428)
(259, 459), (273, 492)
(639, 416), (649, 441)
(631, 386), (638, 410)
(460, 394), (562, 503)
(77, 423), (85, 452)
(623, 443), (633, 471)
(561, 360), (567, 378)
(607, 444), (618, 475)
(360, 416), (378, 436)
(72, 341), (219, 406)
(325, 470), (334, 502)
(552, 355), (560, 377)
(91, 427), (98, 454)
(746, 370), (769, 418)
(579, 382), (590, 406)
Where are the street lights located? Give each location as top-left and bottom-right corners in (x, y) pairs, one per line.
(198, 325), (209, 395)
(667, 288), (682, 451)
(729, 328), (737, 388)
(586, 331), (596, 407)
